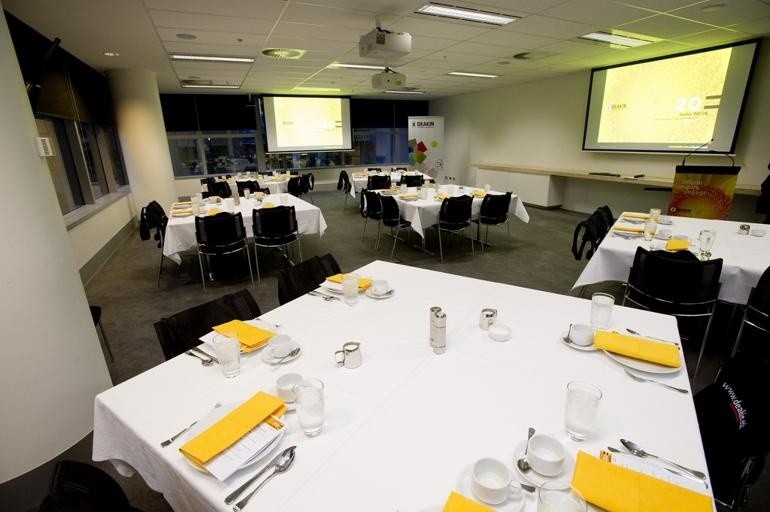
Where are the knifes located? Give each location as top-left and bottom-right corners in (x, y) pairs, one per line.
(192, 346), (219, 364)
(608, 444), (708, 490)
(224, 444), (296, 505)
(626, 328), (679, 345)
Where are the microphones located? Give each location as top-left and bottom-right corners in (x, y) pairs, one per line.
(682, 138), (715, 166)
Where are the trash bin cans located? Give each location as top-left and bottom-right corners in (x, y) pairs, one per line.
(89, 306), (113, 365)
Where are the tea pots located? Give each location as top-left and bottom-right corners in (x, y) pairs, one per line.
(479, 307), (498, 332)
(333, 342), (363, 368)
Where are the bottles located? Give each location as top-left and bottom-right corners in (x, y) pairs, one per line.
(427, 306), (447, 355)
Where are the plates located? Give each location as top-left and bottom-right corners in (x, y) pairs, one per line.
(613, 207), (719, 263)
(456, 484), (527, 512)
(179, 398), (286, 474)
(263, 342), (302, 363)
(604, 348), (684, 375)
(514, 439), (574, 491)
(213, 333), (270, 354)
(560, 331), (602, 352)
(304, 270), (395, 305)
(268, 379), (313, 411)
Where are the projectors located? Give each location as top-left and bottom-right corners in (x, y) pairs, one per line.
(359, 29), (413, 59)
(371, 71), (407, 91)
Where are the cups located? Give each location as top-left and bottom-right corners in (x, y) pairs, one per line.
(471, 457), (522, 504)
(537, 480), (587, 511)
(267, 334), (299, 358)
(212, 331), (242, 379)
(562, 380), (604, 443)
(390, 180), (490, 199)
(570, 323), (594, 344)
(191, 188), (286, 214)
(290, 376), (327, 437)
(277, 372), (303, 403)
(738, 224), (766, 237)
(526, 434), (566, 477)
(589, 292), (614, 331)
(214, 170), (291, 180)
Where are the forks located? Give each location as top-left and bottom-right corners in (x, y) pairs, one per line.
(624, 369), (688, 394)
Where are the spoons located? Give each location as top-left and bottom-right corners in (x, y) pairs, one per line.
(271, 347), (301, 367)
(185, 349), (213, 366)
(233, 449), (296, 511)
(517, 428), (537, 470)
(620, 437), (707, 480)
